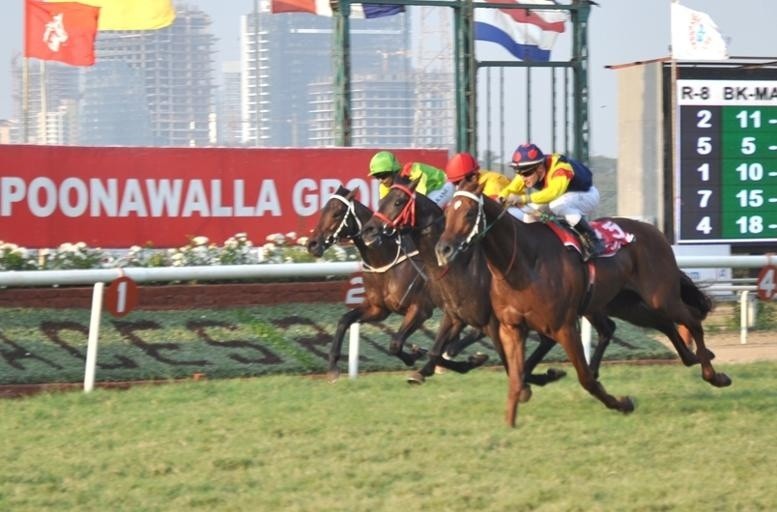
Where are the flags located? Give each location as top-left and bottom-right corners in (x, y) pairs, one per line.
(46, 0), (178, 31)
(471, 0), (572, 62)
(256, 0), (409, 19)
(23, 1), (100, 69)
(669, 2), (731, 62)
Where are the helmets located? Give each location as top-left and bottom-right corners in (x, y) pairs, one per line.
(368, 151), (400, 177)
(509, 143), (546, 168)
(444, 153), (479, 182)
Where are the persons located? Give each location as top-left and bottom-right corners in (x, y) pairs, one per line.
(446, 153), (526, 208)
(367, 150), (456, 211)
(497, 143), (606, 263)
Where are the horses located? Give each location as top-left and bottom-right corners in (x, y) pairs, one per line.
(435, 175), (732, 427)
(303, 185), (489, 380)
(359, 169), (616, 389)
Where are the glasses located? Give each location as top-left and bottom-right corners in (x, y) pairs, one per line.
(374, 173), (389, 179)
(516, 166), (537, 176)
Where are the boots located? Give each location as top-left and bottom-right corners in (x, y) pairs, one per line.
(570, 216), (605, 262)
